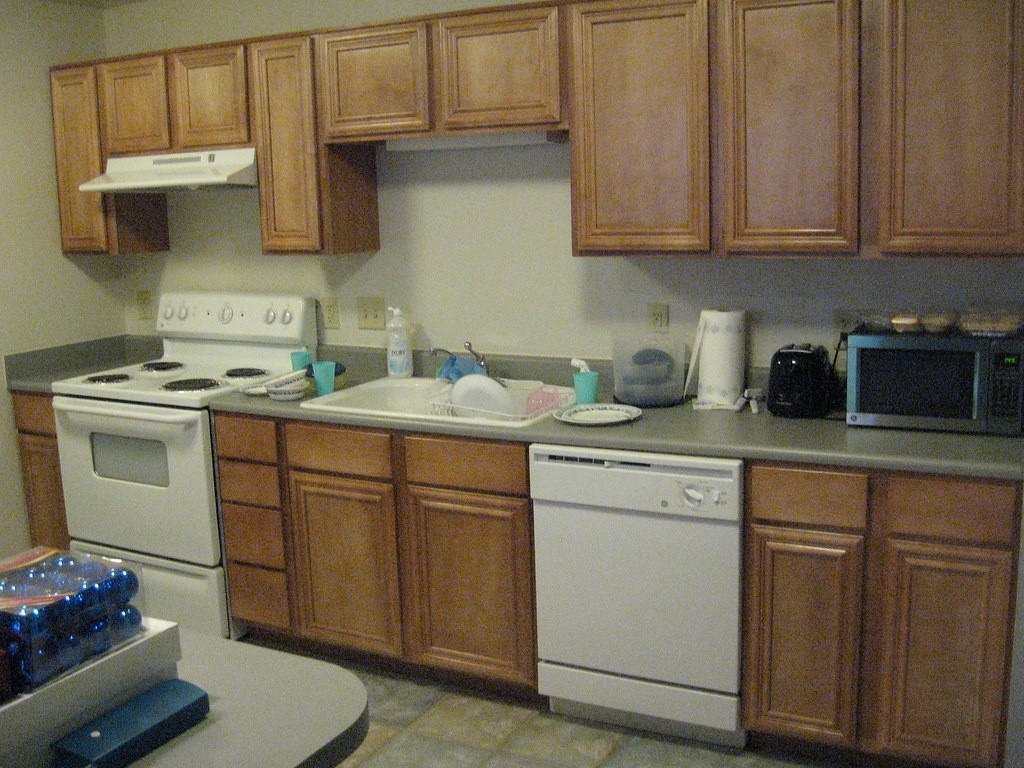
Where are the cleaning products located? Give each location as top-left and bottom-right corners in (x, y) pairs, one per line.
(385, 306), (414, 379)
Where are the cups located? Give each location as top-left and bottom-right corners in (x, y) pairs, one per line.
(312, 362), (336, 397)
(573, 370), (599, 406)
(510, 380), (557, 414)
(290, 351), (313, 378)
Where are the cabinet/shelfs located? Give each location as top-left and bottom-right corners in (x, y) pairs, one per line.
(564, 0), (870, 260)
(314, 0), (570, 140)
(276, 419), (539, 707)
(10, 392), (69, 553)
(96, 40), (254, 158)
(210, 410), (296, 636)
(740, 460), (1024, 768)
(244, 29), (380, 255)
(48, 60), (171, 258)
(875, 0), (1024, 264)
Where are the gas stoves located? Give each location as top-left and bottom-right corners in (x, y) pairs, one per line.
(51, 293), (318, 407)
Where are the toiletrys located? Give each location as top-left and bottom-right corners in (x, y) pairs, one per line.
(570, 358), (592, 373)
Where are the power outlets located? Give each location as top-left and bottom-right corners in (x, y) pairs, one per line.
(646, 302), (670, 334)
(320, 296), (341, 330)
(136, 290), (154, 321)
(833, 309), (857, 351)
(355, 296), (387, 329)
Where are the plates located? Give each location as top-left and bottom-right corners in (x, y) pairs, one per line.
(451, 374), (513, 416)
(239, 385), (267, 397)
(553, 404), (642, 424)
(264, 369), (310, 402)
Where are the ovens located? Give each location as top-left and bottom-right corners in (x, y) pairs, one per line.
(51, 393), (250, 640)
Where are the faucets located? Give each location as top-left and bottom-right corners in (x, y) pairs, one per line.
(427, 340), (490, 377)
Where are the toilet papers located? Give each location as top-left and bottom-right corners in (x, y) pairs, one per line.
(683, 302), (747, 405)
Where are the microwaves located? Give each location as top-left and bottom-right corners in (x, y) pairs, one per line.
(844, 328), (1024, 438)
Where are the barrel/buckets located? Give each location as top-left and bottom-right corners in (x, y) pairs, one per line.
(612, 326), (685, 410)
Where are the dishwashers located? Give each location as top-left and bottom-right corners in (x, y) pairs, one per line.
(524, 442), (748, 748)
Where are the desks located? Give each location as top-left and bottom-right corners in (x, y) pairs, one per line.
(124, 629), (371, 768)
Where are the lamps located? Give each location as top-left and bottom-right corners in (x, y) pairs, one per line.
(374, 129), (567, 152)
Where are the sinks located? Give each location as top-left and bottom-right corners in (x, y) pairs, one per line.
(299, 374), (579, 429)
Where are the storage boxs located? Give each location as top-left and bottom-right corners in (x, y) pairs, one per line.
(0, 616), (184, 768)
(47, 677), (211, 768)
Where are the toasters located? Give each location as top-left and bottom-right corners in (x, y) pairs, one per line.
(767, 343), (841, 418)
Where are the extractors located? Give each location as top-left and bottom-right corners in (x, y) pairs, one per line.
(77, 148), (256, 193)
